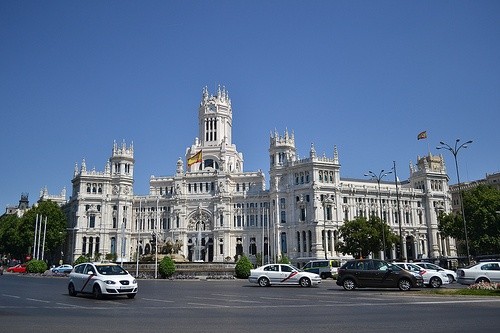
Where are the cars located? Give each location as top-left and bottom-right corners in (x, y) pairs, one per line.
(409, 262), (457, 284)
(378, 262), (450, 288)
(0, 261), (5, 275)
(6, 263), (27, 273)
(68, 262), (138, 300)
(248, 264), (322, 288)
(456, 261), (500, 285)
(52, 264), (73, 274)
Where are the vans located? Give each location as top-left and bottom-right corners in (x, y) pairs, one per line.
(298, 259), (342, 280)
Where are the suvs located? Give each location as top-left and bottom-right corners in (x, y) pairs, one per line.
(336, 259), (424, 291)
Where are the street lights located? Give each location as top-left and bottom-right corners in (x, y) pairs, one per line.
(363, 170), (393, 260)
(436, 138), (473, 267)
